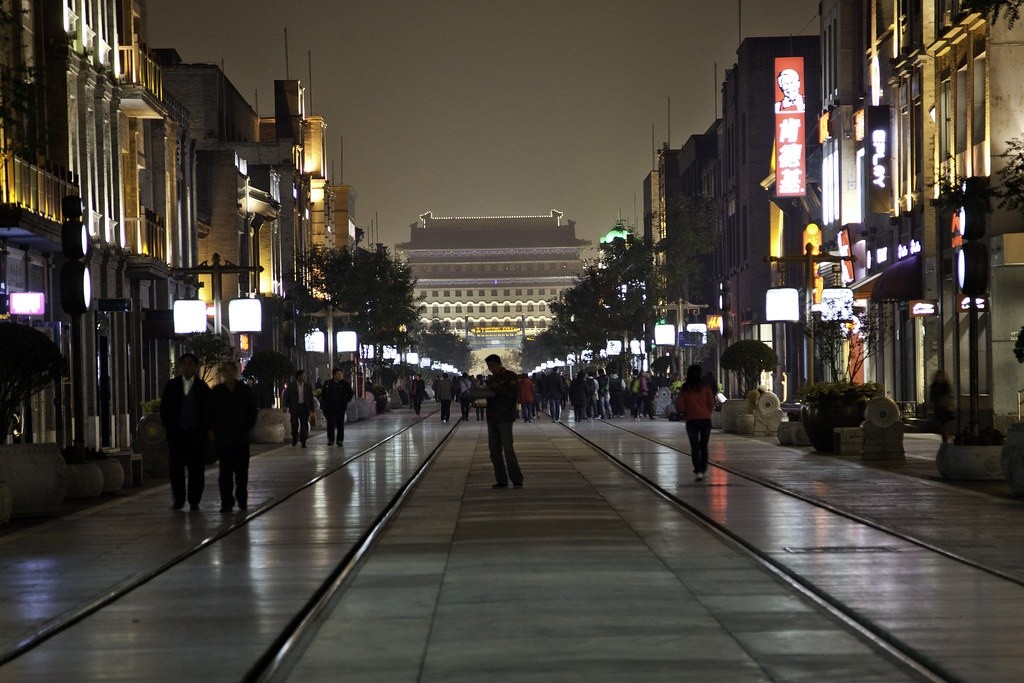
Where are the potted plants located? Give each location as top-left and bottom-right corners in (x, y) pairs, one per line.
(801, 380), (882, 453)
(937, 424), (1006, 481)
(63, 444), (125, 496)
(712, 338), (780, 430)
(131, 398), (165, 452)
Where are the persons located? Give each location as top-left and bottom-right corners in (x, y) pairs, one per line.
(702, 371), (718, 394)
(160, 353), (257, 512)
(432, 373), (491, 423)
(282, 370), (314, 448)
(680, 365), (714, 480)
(315, 378), (322, 388)
(407, 374), (425, 415)
(519, 366), (678, 423)
(321, 368), (354, 447)
(477, 354), (523, 489)
(366, 377), (371, 384)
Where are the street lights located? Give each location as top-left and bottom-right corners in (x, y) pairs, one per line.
(717, 280), (729, 399)
(59, 196), (92, 463)
(957, 179), (990, 444)
(297, 303), (358, 379)
(165, 251), (264, 383)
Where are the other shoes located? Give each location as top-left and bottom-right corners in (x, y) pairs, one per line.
(337, 440), (343, 446)
(328, 437), (335, 445)
(300, 439), (306, 448)
(513, 475), (523, 489)
(236, 494), (247, 510)
(171, 497), (185, 510)
(492, 483), (508, 488)
(694, 472), (703, 481)
(188, 500), (199, 512)
(220, 498), (234, 512)
(292, 433), (299, 445)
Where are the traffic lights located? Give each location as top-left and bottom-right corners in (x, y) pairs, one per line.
(659, 318), (666, 325)
